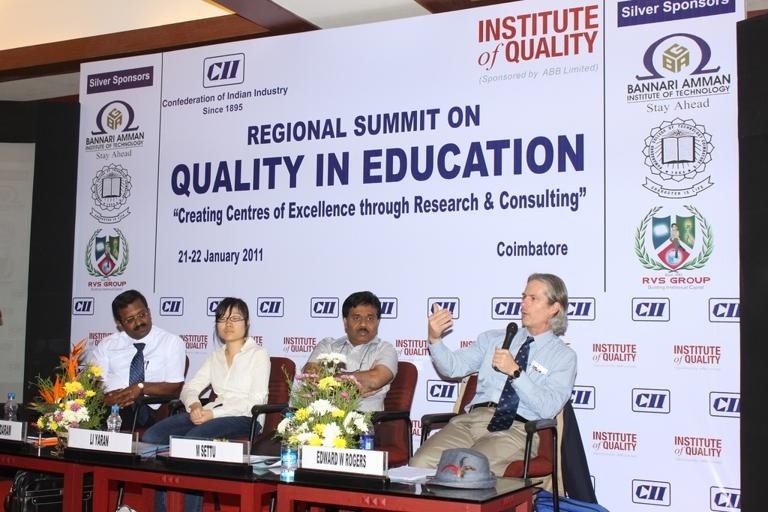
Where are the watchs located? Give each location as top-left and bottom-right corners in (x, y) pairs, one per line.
(510, 365), (523, 379)
(138, 382), (144, 395)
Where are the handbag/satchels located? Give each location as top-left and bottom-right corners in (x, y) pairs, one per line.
(5, 469), (93, 512)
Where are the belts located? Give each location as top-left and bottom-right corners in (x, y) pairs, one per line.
(468, 403), (525, 423)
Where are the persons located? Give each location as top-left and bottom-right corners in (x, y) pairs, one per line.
(251, 291), (398, 457)
(141, 298), (271, 512)
(80, 289), (187, 431)
(409, 273), (577, 479)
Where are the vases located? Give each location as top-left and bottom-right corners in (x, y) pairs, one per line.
(50, 431), (67, 457)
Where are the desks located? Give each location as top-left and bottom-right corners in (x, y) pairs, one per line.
(264, 467), (543, 512)
(1, 436), (162, 512)
(73, 450), (301, 512)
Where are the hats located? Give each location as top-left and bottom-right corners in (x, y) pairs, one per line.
(427, 448), (497, 488)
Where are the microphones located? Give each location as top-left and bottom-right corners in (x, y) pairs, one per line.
(495, 322), (518, 372)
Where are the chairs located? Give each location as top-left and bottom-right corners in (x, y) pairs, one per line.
(155, 358), (295, 512)
(269, 361), (418, 511)
(420, 372), (564, 512)
(54, 354), (189, 512)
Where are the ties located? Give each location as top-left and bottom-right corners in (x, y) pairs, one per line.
(129, 343), (150, 426)
(487, 336), (534, 432)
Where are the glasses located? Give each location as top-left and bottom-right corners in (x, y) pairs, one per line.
(121, 311), (149, 324)
(216, 315), (246, 322)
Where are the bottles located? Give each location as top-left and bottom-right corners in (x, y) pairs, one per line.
(280, 468), (295, 484)
(359, 426), (374, 450)
(3, 392), (19, 421)
(281, 413), (298, 469)
(107, 406), (122, 433)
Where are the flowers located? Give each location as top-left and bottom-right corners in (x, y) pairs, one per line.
(270, 358), (379, 450)
(25, 336), (108, 435)
